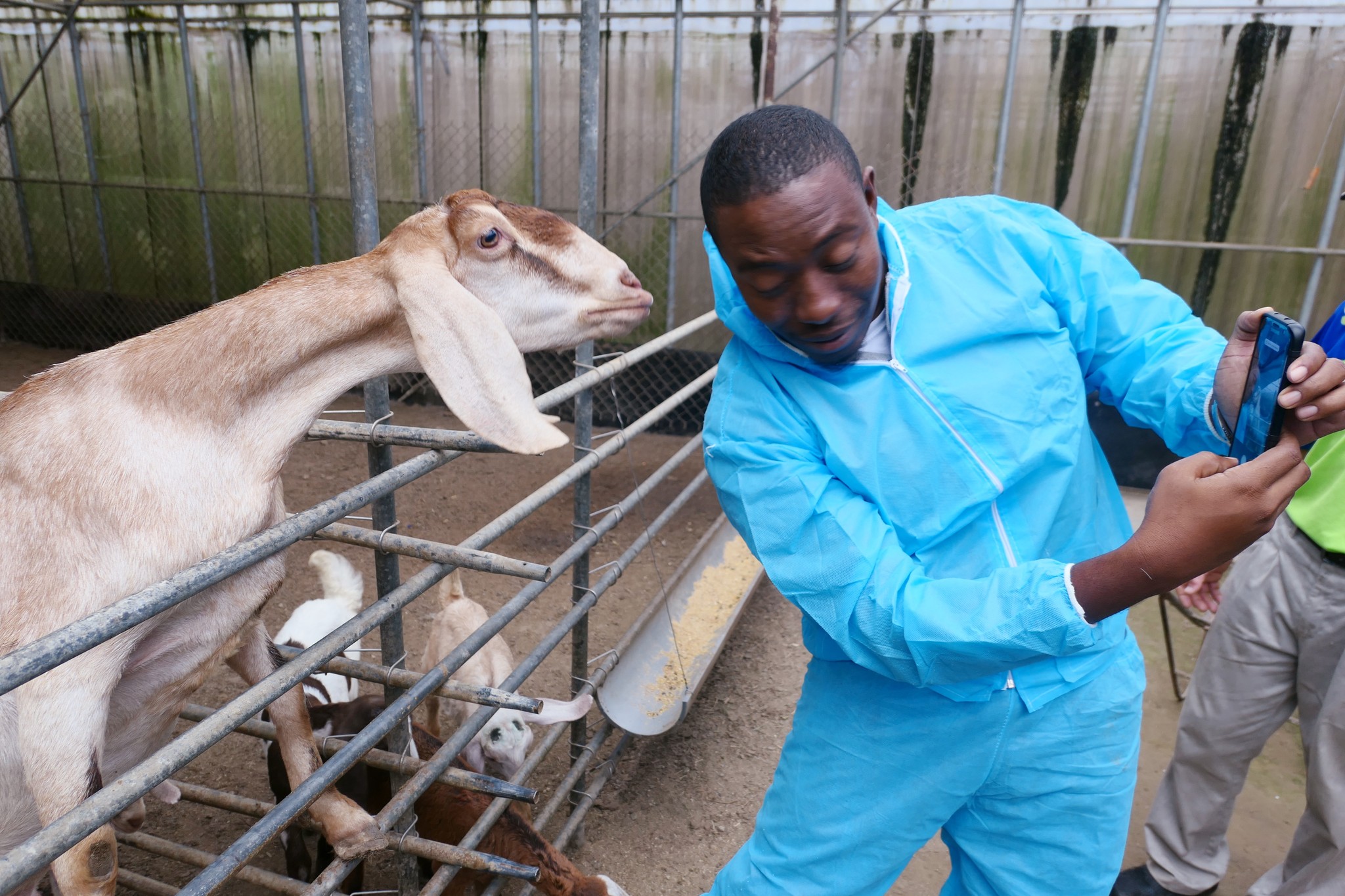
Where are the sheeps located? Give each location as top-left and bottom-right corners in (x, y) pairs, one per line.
(0, 185), (659, 896)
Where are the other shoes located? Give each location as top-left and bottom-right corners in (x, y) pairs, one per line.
(1109, 861), (1220, 896)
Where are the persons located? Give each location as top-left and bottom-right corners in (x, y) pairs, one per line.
(1104, 296), (1345, 896)
(698, 106), (1345, 896)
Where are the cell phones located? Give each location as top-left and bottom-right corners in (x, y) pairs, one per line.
(1229, 312), (1306, 466)
